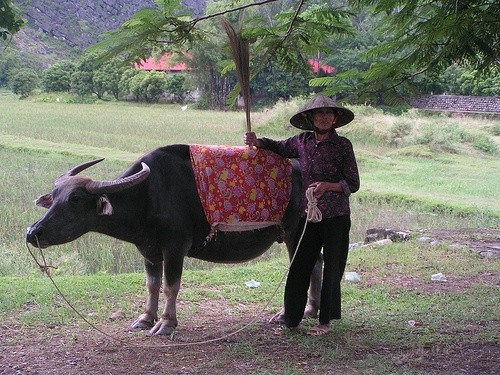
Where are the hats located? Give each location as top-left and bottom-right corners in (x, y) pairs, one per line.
(290, 93), (354, 131)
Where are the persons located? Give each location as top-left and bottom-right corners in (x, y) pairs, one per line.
(244, 94), (360, 336)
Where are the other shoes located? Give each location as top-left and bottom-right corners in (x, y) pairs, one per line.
(274, 327), (290, 336)
(307, 324), (331, 337)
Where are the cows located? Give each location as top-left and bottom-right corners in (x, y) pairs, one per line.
(25, 143), (323, 335)
